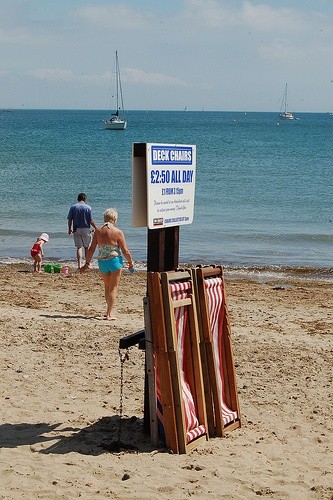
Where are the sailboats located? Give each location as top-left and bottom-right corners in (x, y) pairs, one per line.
(103, 51), (127, 131)
(278, 81), (295, 119)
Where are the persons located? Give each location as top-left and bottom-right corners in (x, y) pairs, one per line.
(30, 233), (49, 273)
(79, 208), (137, 321)
(67, 193), (100, 271)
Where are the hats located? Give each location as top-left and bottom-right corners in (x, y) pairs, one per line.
(38, 233), (48, 242)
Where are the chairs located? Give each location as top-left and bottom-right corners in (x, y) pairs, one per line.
(143, 264), (242, 455)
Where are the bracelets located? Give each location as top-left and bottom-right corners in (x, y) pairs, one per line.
(128, 260), (134, 265)
(86, 262), (89, 265)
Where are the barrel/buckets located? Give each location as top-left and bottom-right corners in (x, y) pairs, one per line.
(53, 264), (62, 273)
(60, 266), (69, 274)
(43, 264), (52, 274)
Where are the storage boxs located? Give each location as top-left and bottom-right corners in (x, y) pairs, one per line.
(44, 264), (69, 274)
